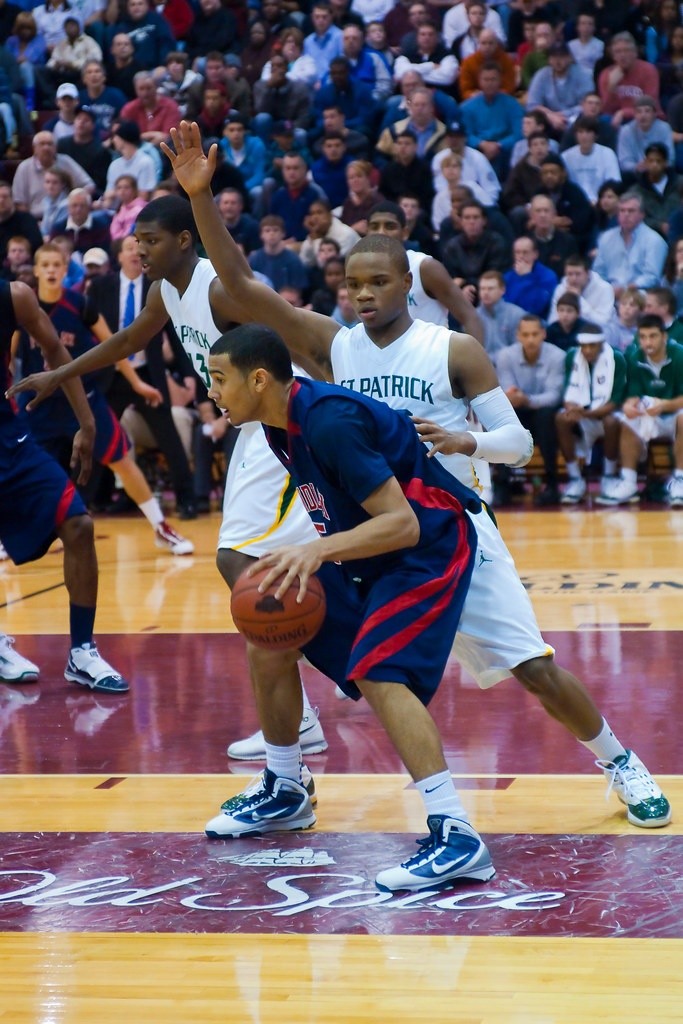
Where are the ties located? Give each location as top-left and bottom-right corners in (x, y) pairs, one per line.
(123, 282), (134, 361)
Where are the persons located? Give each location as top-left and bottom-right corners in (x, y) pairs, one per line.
(159, 119), (673, 827)
(204, 322), (497, 894)
(1, 0), (683, 758)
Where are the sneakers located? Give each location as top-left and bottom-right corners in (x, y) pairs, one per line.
(228, 706), (329, 759)
(64, 641), (130, 693)
(0, 632), (40, 681)
(493, 475), (683, 506)
(65, 695), (130, 736)
(220, 764), (316, 812)
(153, 523), (193, 554)
(205, 768), (315, 838)
(594, 749), (672, 827)
(374, 813), (494, 891)
(157, 558), (194, 574)
(0, 682), (41, 723)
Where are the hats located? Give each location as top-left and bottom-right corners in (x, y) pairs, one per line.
(447, 121), (464, 133)
(75, 104), (95, 121)
(83, 248), (109, 266)
(116, 123), (138, 143)
(57, 83), (79, 98)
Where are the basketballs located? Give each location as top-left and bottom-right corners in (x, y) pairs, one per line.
(231, 560), (326, 652)
